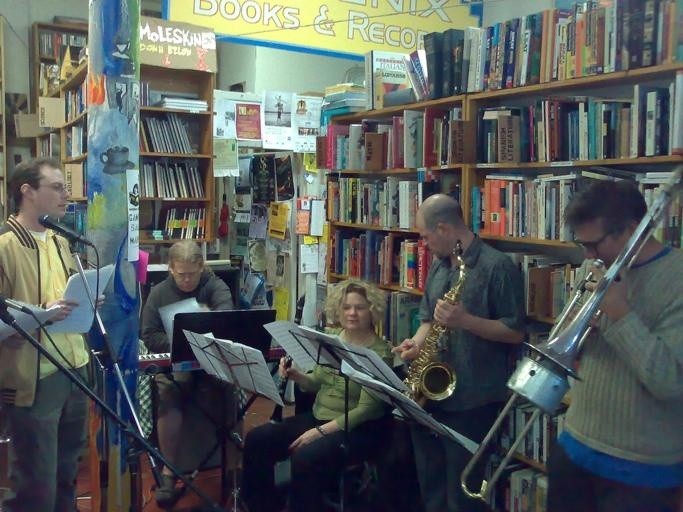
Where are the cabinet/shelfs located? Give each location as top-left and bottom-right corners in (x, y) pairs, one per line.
(30, 58), (88, 268)
(0, 13), (6, 230)
(136, 63), (214, 244)
(327, 94), (464, 378)
(465, 61), (683, 512)
(29, 20), (89, 113)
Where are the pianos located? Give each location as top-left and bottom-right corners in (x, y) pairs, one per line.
(139, 346), (287, 370)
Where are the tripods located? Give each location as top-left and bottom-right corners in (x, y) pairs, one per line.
(182, 338), (260, 512)
(351, 375), (448, 512)
(286, 328), (408, 512)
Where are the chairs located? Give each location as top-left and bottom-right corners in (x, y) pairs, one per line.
(141, 267), (239, 329)
(295, 361), (405, 512)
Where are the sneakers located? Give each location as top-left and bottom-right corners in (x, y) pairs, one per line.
(155, 473), (178, 509)
(226, 468), (243, 490)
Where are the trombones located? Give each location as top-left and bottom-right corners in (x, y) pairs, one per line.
(460, 166), (683, 501)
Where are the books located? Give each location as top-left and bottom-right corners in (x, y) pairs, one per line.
(498, 250), (605, 324)
(507, 330), (577, 403)
(331, 232), (436, 293)
(60, 202), (87, 255)
(330, 108), (462, 169)
(64, 159), (87, 198)
(505, 468), (549, 512)
(39, 133), (61, 164)
(62, 74), (87, 123)
(152, 207), (206, 239)
(40, 63), (83, 95)
(63, 121), (87, 157)
(472, 167), (683, 243)
(35, 107), (62, 130)
(138, 161), (205, 198)
(325, 173), (461, 230)
(139, 80), (209, 111)
(326, 283), (421, 347)
(474, 72), (682, 164)
(499, 401), (568, 467)
(139, 112), (194, 153)
(38, 28), (87, 59)
(322, 83), (363, 114)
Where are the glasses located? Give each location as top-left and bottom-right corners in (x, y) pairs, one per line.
(577, 227), (617, 259)
(40, 182), (68, 192)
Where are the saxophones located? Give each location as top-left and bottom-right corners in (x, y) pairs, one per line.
(393, 238), (469, 421)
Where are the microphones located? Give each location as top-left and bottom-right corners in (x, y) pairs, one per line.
(0, 289), (33, 317)
(37, 213), (93, 250)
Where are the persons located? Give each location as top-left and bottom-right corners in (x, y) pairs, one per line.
(1, 156), (105, 512)
(140, 241), (234, 508)
(391, 193), (526, 512)
(265, 91), (288, 124)
(241, 279), (395, 512)
(103, 32), (136, 76)
(547, 179), (683, 512)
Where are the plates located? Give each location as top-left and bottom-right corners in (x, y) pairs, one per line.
(103, 161), (135, 174)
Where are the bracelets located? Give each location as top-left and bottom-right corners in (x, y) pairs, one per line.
(315, 426), (328, 436)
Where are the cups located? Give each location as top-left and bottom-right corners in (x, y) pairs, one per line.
(99, 146), (129, 167)
